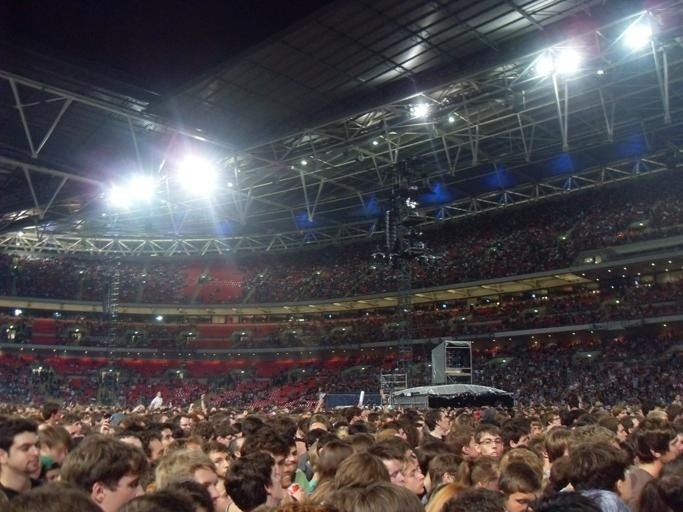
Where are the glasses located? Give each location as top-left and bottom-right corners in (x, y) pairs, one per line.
(479, 440), (502, 445)
